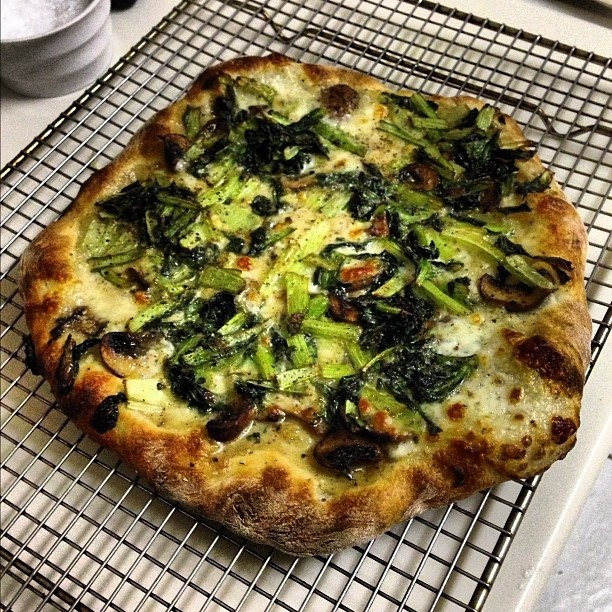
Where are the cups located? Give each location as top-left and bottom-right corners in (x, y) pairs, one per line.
(1, 1), (116, 100)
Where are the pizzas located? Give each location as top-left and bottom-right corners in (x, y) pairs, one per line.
(19, 57), (591, 555)
